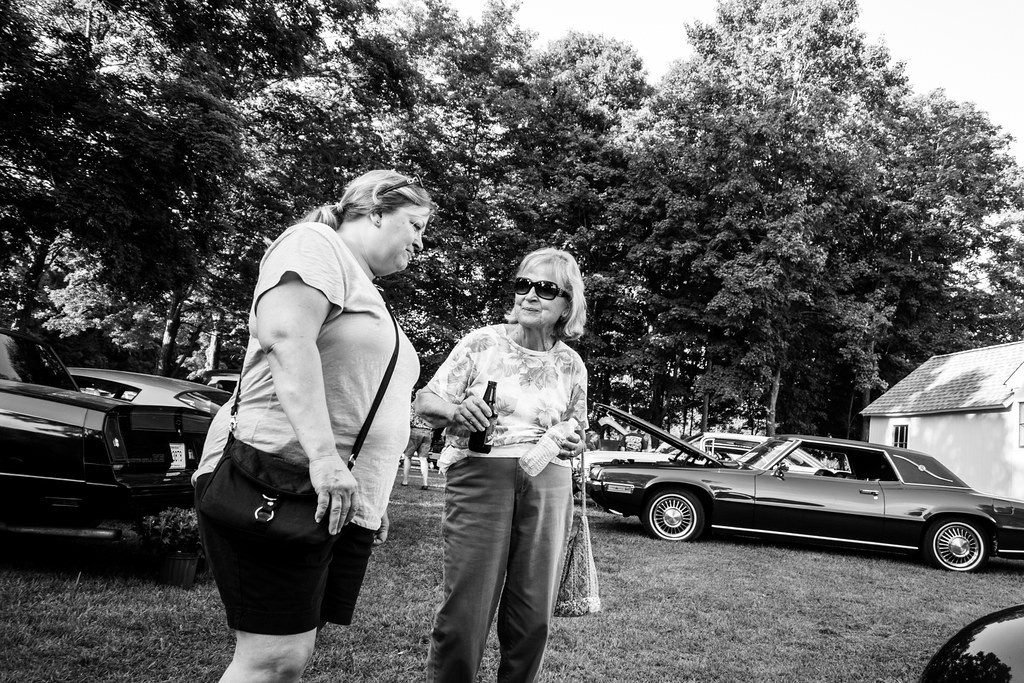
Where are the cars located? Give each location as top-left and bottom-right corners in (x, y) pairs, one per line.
(65, 365), (241, 418)
(585, 401), (1024, 571)
(564, 430), (848, 494)
(0, 328), (228, 585)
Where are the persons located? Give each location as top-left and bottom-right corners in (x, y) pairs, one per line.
(620, 424), (646, 452)
(191, 170), (430, 683)
(401, 389), (431, 490)
(416, 248), (587, 683)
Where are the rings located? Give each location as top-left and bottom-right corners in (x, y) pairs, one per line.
(569, 448), (577, 458)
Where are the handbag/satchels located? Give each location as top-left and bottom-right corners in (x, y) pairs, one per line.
(552, 513), (601, 616)
(195, 431), (343, 547)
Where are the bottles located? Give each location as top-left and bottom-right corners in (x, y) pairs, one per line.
(467, 380), (498, 455)
(520, 418), (578, 477)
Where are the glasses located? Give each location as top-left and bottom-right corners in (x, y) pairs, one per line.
(514, 276), (572, 301)
